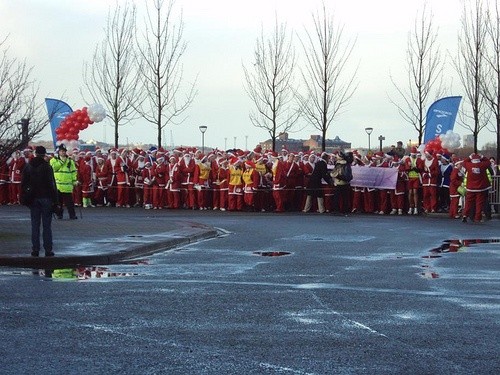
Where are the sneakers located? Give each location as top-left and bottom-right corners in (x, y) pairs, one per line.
(73, 202), (448, 216)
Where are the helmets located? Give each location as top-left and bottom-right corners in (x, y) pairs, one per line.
(58, 144), (67, 151)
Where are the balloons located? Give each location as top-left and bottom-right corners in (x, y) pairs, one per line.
(418, 130), (462, 154)
(54, 103), (107, 153)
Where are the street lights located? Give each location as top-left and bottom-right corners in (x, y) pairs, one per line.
(199, 126), (207, 154)
(365, 128), (373, 150)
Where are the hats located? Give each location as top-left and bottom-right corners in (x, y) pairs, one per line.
(454, 157), (464, 165)
(35, 146), (45, 155)
(12, 146), (450, 165)
(472, 154), (480, 160)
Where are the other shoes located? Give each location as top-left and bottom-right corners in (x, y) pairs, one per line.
(0, 200), (20, 206)
(45, 250), (54, 256)
(487, 216), (492, 220)
(462, 215), (467, 223)
(455, 215), (460, 219)
(71, 215), (77, 219)
(58, 216), (63, 219)
(31, 251), (39, 256)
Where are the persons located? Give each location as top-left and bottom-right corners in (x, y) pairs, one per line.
(0, 143), (500, 256)
